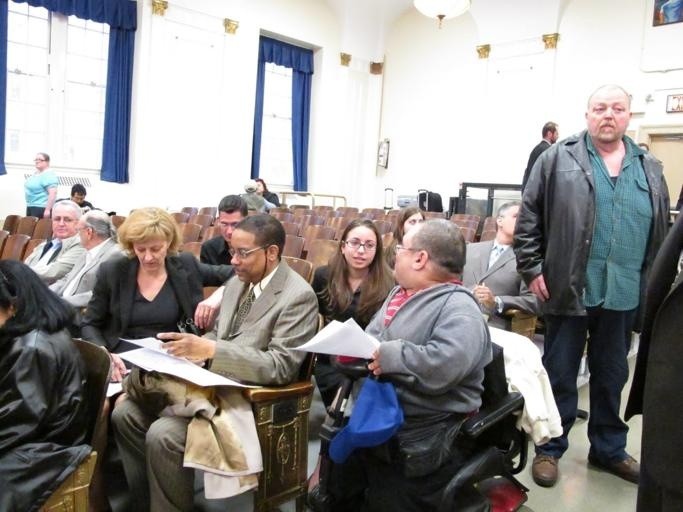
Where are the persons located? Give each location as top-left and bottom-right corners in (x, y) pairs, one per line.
(462, 202), (538, 331)
(253, 181), (280, 206)
(386, 206), (426, 270)
(238, 179), (276, 213)
(48, 210), (121, 308)
(25, 153), (59, 218)
(308, 219), (493, 511)
(311, 220), (398, 406)
(1, 259), (94, 512)
(55, 184), (93, 215)
(24, 200), (85, 288)
(521, 123), (559, 198)
(80, 208), (236, 512)
(623, 185), (683, 512)
(200, 196), (248, 265)
(514, 84), (671, 488)
(112, 215), (320, 511)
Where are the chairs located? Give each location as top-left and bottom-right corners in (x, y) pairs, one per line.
(303, 226), (336, 256)
(240, 314), (322, 512)
(280, 220), (300, 237)
(451, 214), (480, 223)
(485, 217), (497, 224)
(281, 255), (313, 284)
(200, 285), (219, 299)
(292, 204), (404, 226)
(32, 217), (54, 239)
(305, 238), (340, 268)
(16, 216), (38, 238)
(191, 214), (213, 238)
(27, 337), (114, 512)
(204, 226), (217, 243)
(0, 227), (10, 255)
(177, 221), (202, 244)
(271, 212), (296, 225)
(184, 241), (204, 260)
(2, 215), (20, 235)
(457, 226), (476, 245)
(494, 298), (536, 345)
(483, 223), (498, 232)
(170, 212), (190, 224)
(110, 214), (126, 230)
(283, 234), (305, 260)
(199, 208), (217, 220)
(453, 219), (479, 232)
(22, 235), (45, 261)
(0, 232), (29, 262)
(480, 230), (497, 241)
(181, 207), (197, 218)
(373, 219), (392, 233)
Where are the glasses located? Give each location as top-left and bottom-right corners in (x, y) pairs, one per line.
(217, 219), (239, 228)
(341, 239), (377, 250)
(228, 242), (272, 258)
(34, 159), (47, 161)
(395, 244), (422, 254)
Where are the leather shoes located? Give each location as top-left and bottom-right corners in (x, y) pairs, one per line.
(532, 453), (560, 488)
(588, 453), (641, 484)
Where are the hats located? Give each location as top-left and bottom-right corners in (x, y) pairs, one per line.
(243, 180), (259, 193)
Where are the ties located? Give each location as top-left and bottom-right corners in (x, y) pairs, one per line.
(231, 287), (256, 335)
